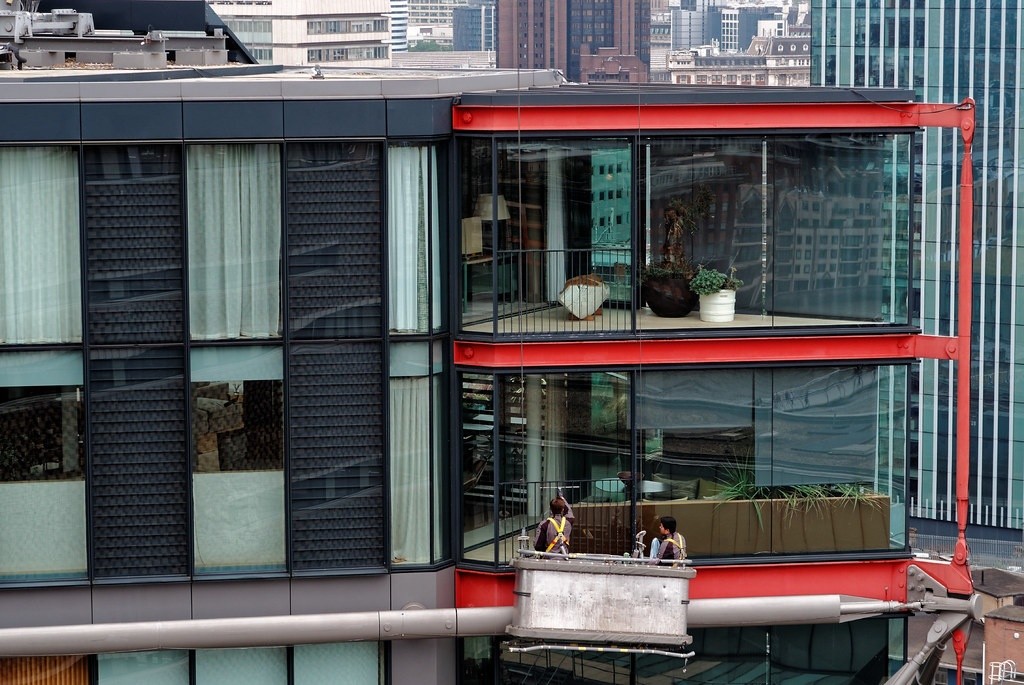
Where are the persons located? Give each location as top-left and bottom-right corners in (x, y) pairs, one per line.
(531, 492), (575, 561)
(649, 517), (687, 571)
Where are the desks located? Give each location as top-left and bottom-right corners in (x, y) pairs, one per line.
(462, 250), (515, 313)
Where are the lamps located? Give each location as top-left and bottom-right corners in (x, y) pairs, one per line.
(473, 193), (511, 252)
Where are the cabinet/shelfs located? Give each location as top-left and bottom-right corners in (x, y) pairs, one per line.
(476, 146), (547, 302)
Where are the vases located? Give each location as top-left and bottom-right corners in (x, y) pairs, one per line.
(604, 281), (631, 302)
(558, 275), (611, 320)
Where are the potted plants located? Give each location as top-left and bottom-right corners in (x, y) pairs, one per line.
(689, 247), (745, 322)
(642, 197), (716, 319)
(623, 265), (632, 285)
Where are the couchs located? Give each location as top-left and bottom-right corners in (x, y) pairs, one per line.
(196, 382), (243, 435)
